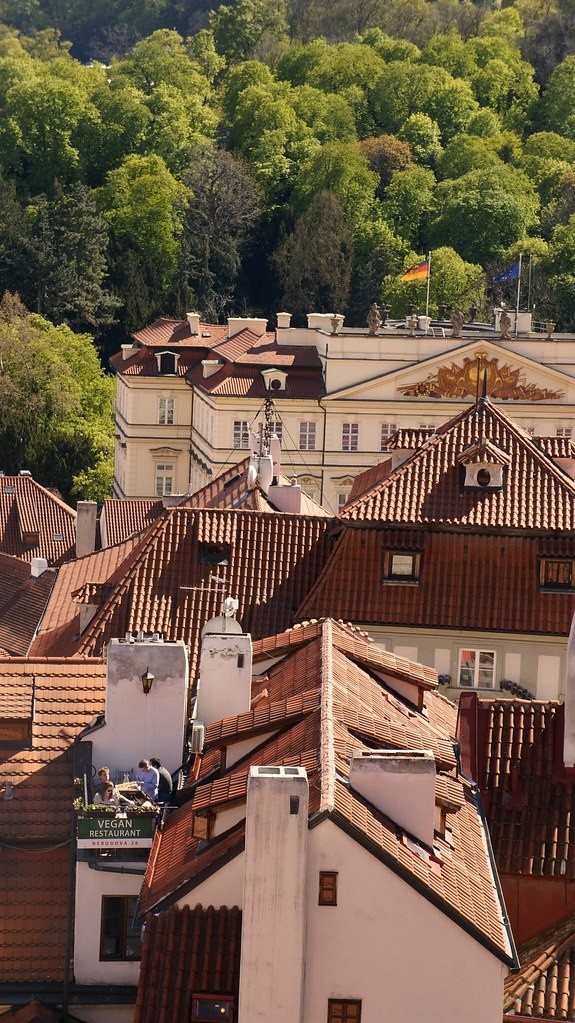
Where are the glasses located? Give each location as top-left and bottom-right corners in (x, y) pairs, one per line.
(106, 790), (112, 792)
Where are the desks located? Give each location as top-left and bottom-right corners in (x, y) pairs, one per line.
(115, 781), (142, 800)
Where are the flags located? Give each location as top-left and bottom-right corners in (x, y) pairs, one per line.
(401, 260), (429, 281)
(492, 260), (519, 283)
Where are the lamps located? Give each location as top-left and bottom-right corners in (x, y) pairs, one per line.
(142, 666), (155, 695)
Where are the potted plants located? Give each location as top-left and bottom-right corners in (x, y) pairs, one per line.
(73, 796), (118, 818)
(125, 808), (157, 818)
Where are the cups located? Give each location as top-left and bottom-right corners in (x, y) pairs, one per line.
(123, 778), (129, 784)
(124, 771), (128, 778)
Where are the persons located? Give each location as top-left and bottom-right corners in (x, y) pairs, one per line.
(89, 766), (157, 832)
(136, 759), (161, 803)
(498, 308), (512, 337)
(450, 307), (465, 335)
(365, 302), (381, 333)
(149, 757), (172, 802)
